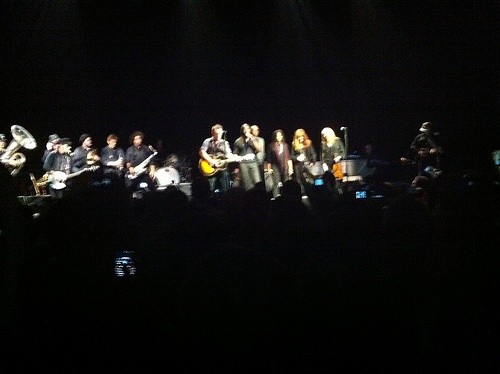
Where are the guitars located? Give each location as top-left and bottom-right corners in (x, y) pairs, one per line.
(199, 153), (254, 175)
(50, 163), (98, 190)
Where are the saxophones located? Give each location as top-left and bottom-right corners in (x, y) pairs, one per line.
(125, 144), (160, 181)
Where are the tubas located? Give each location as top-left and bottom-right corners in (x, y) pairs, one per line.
(2, 121), (39, 176)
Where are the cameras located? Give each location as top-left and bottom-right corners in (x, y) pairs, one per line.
(355, 190), (369, 199)
(315, 179), (324, 186)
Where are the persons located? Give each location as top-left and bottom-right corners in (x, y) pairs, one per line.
(411, 122), (442, 174)
(0, 124), (500, 374)
